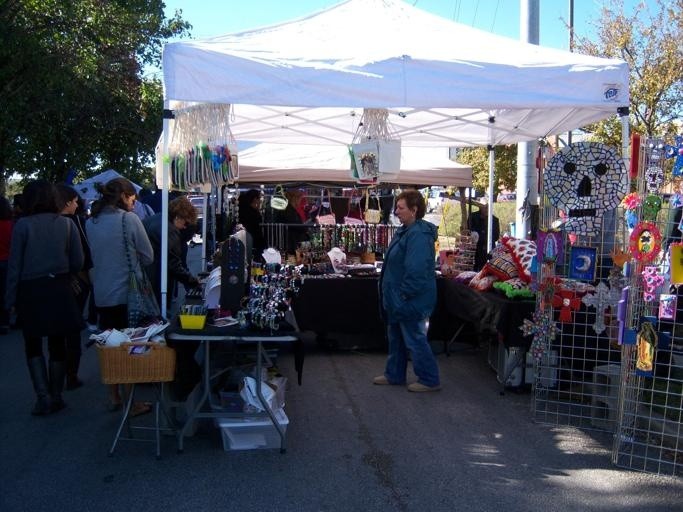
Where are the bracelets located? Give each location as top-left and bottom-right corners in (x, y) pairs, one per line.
(247, 263), (303, 332)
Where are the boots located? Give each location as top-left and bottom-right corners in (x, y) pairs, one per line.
(26, 355), (53, 416)
(48, 359), (67, 414)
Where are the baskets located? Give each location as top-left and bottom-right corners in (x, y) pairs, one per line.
(96, 342), (176, 384)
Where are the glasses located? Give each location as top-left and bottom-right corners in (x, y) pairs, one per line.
(130, 196), (137, 207)
(184, 220), (190, 226)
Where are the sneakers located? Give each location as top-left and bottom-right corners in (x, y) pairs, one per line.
(408, 383), (441, 392)
(66, 379), (84, 390)
(373, 376), (390, 385)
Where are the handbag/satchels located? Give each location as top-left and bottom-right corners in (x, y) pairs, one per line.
(270, 184), (288, 210)
(348, 109), (402, 185)
(364, 209), (380, 224)
(343, 217), (365, 233)
(168, 103), (238, 190)
(315, 213), (335, 226)
(65, 266), (90, 298)
(127, 287), (161, 329)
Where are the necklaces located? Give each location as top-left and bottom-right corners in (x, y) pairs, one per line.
(314, 223), (394, 255)
(228, 235), (240, 286)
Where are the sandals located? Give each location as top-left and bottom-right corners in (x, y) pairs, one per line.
(111, 399), (122, 411)
(129, 405), (152, 417)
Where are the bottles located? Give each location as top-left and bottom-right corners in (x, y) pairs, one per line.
(216, 305), (221, 319)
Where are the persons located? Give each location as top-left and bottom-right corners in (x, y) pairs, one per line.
(238, 190), (263, 262)
(467, 197), (500, 272)
(278, 191), (309, 255)
(373, 190), (441, 392)
(661, 175), (683, 252)
(0, 178), (202, 417)
